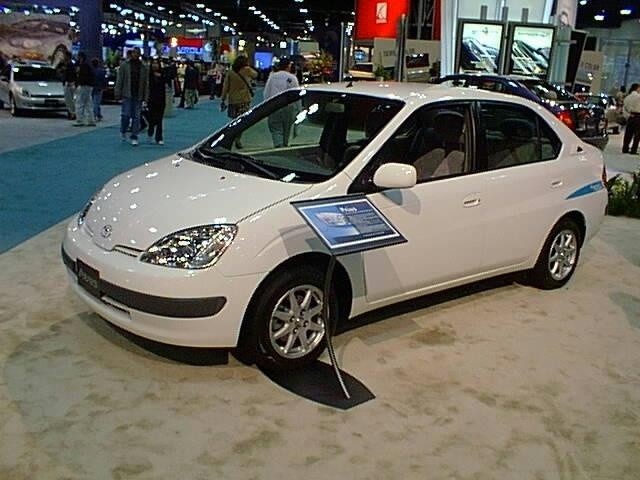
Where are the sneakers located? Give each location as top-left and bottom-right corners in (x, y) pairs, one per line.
(120, 125), (164, 146)
(72, 114), (103, 127)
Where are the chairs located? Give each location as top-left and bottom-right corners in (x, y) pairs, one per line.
(361, 110), (538, 183)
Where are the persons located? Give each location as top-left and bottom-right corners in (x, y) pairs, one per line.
(616, 85), (630, 111)
(54, 46), (303, 149)
(557, 10), (573, 29)
(622, 83), (640, 156)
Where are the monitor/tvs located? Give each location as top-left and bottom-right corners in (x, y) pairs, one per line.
(355, 50), (365, 60)
(405, 53), (430, 71)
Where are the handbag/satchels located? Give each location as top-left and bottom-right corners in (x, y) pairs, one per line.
(248, 88), (257, 97)
(294, 119), (301, 137)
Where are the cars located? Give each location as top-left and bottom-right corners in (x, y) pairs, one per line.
(1, 58), (77, 120)
(61, 81), (609, 371)
(426, 74), (620, 149)
